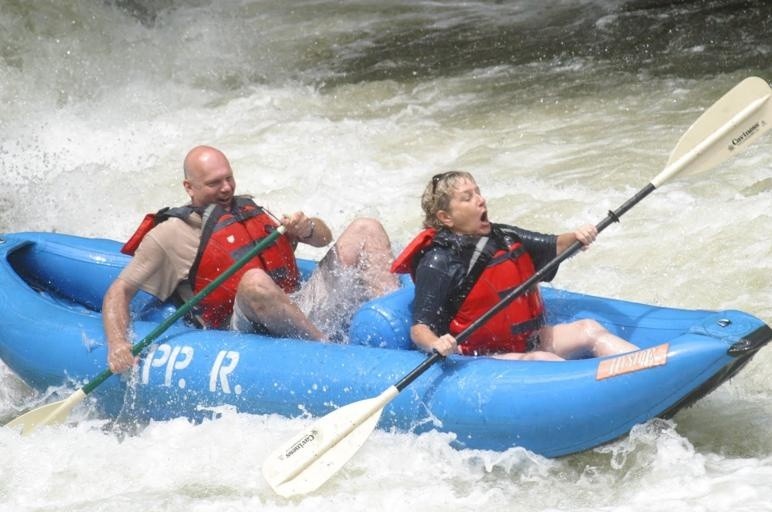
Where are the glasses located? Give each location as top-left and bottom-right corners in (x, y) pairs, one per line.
(432, 170), (456, 201)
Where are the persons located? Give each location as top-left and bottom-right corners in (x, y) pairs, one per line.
(101, 145), (403, 375)
(390, 170), (642, 363)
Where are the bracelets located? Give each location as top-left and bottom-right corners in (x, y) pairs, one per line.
(305, 217), (316, 238)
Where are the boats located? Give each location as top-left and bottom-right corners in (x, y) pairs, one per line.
(0, 232), (772, 458)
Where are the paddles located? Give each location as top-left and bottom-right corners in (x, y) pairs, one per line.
(261, 76), (771, 496)
(4, 225), (288, 430)
